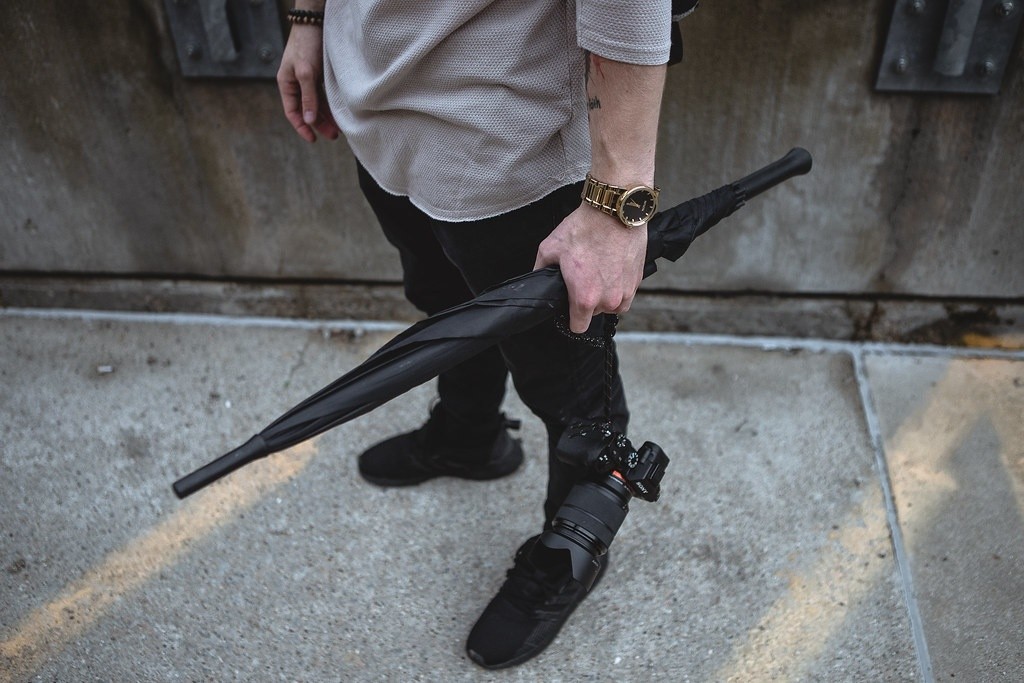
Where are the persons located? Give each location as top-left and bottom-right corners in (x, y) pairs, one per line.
(276, 0), (671, 670)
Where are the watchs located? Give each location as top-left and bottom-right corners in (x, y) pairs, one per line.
(580, 171), (660, 228)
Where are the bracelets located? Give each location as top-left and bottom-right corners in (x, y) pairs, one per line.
(288, 7), (324, 18)
(286, 15), (323, 26)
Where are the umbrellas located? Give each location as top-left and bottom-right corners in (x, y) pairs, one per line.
(172, 147), (812, 498)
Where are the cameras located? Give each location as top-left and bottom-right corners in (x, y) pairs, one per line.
(516, 415), (673, 601)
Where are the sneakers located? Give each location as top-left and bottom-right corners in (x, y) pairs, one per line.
(357, 394), (523, 486)
(465, 524), (609, 670)
